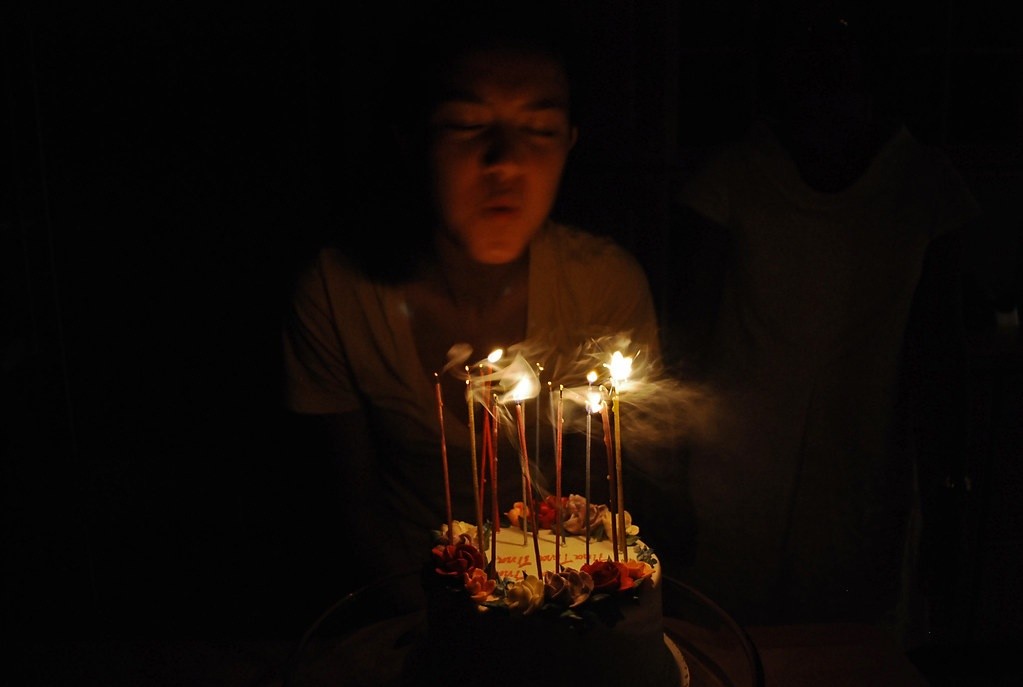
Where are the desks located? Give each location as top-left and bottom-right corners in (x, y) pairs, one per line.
(285, 559), (767, 687)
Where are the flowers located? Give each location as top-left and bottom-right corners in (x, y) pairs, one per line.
(436, 493), (653, 623)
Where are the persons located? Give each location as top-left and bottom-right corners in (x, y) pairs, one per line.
(661, 0), (981, 687)
(279, 0), (699, 619)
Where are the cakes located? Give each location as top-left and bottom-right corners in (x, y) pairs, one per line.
(425, 494), (666, 687)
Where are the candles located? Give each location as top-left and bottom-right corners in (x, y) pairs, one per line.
(432, 348), (639, 576)
